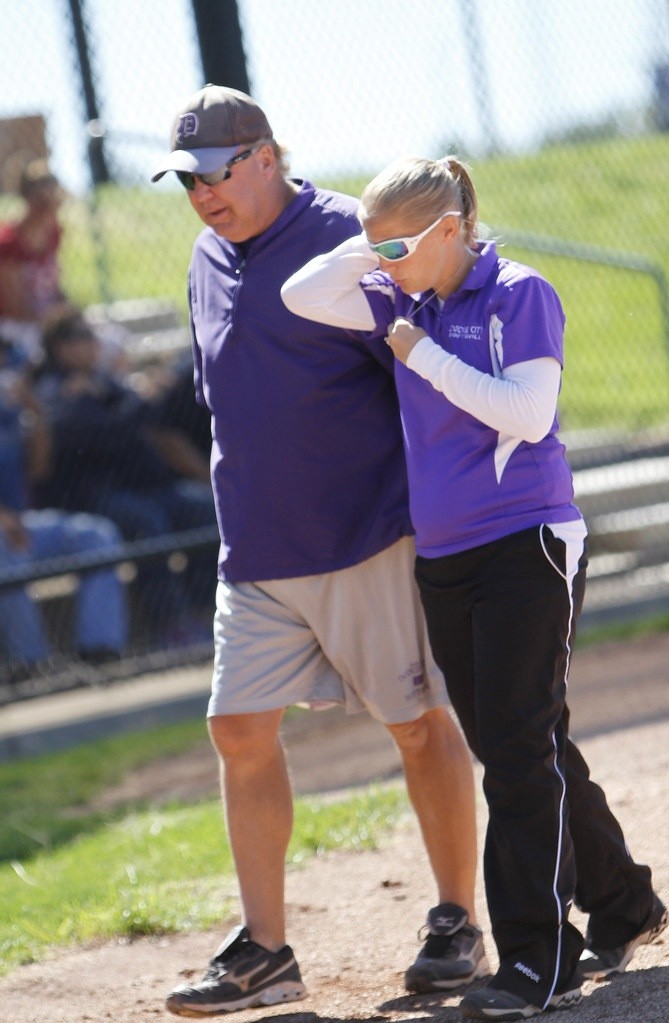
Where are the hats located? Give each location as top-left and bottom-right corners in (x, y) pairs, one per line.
(151, 82), (272, 183)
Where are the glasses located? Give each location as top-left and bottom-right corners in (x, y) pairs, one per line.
(175, 148), (256, 191)
(361, 211), (462, 262)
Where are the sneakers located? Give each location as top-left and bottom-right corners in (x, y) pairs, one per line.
(572, 893), (669, 979)
(405, 904), (491, 992)
(459, 974), (583, 1022)
(166, 924), (307, 1015)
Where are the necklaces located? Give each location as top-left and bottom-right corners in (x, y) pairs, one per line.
(405, 248), (473, 322)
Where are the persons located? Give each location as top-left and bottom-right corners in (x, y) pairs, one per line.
(281, 154), (668, 1023)
(0, 162), (220, 681)
(151, 84), (489, 1016)
